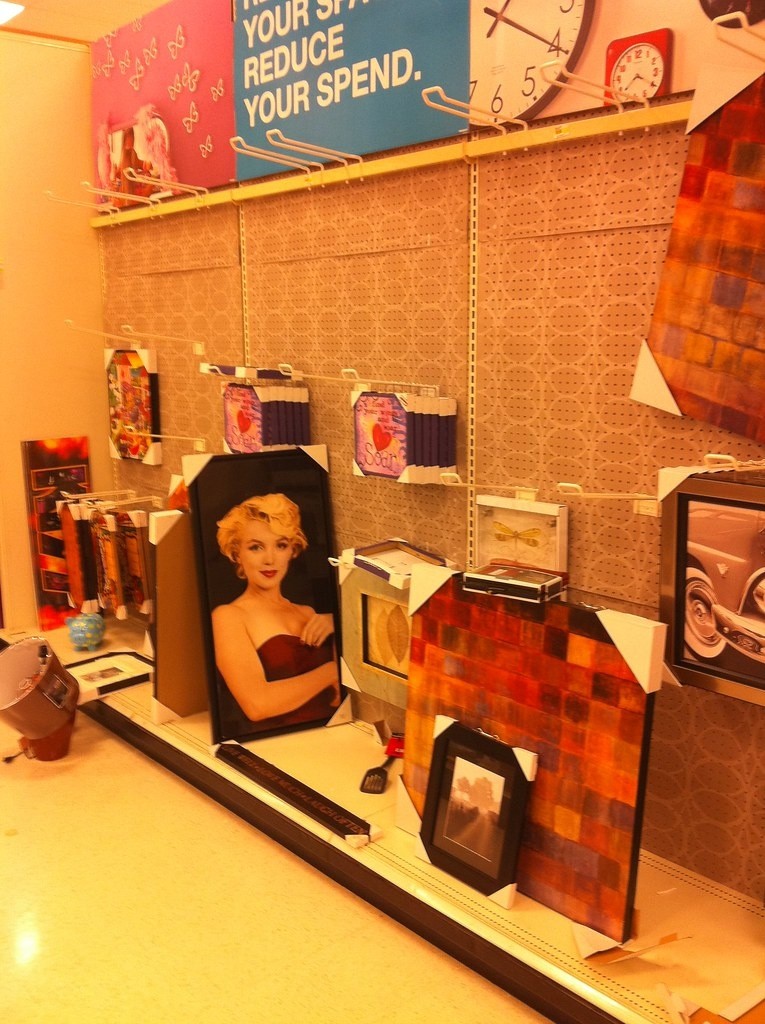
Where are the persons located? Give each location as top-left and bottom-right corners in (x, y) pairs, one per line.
(212, 495), (340, 738)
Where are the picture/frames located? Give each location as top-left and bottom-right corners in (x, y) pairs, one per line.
(104, 348), (163, 466)
(179, 443), (355, 744)
(342, 537), (457, 591)
(340, 561), (412, 709)
(349, 390), (457, 486)
(660, 477), (765, 707)
(412, 714), (539, 910)
(64, 646), (154, 706)
(220, 382), (310, 455)
(56, 497), (156, 621)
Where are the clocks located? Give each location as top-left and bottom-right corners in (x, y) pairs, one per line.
(470, 0), (596, 129)
(603, 28), (673, 106)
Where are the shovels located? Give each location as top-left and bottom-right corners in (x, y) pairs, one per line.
(359, 756), (397, 793)
